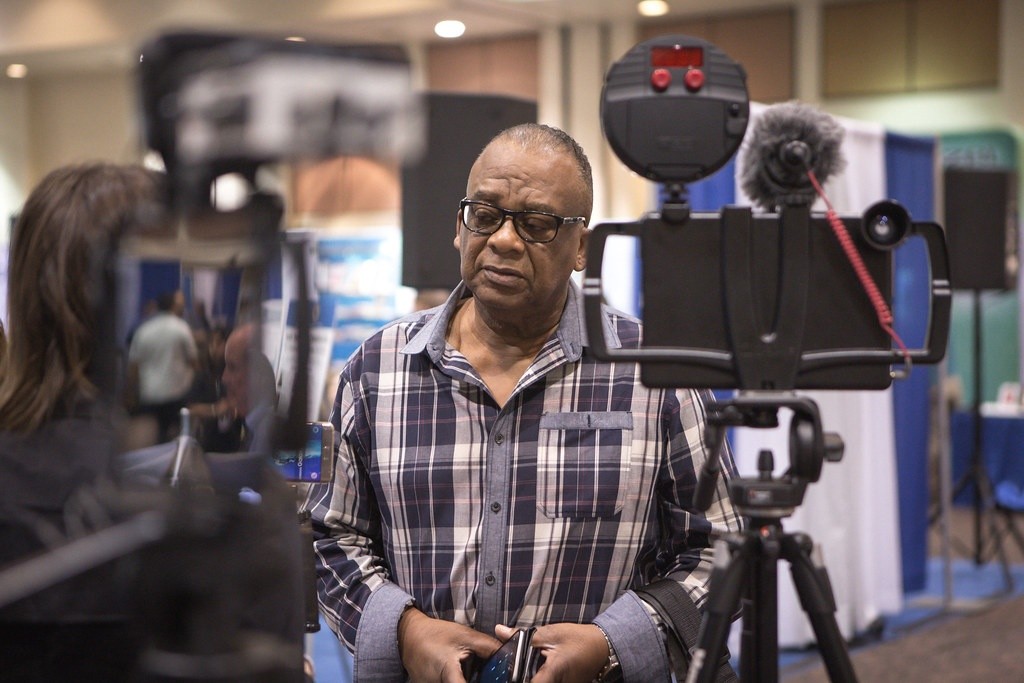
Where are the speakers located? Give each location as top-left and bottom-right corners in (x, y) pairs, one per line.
(400, 90), (539, 289)
(945, 169), (1019, 288)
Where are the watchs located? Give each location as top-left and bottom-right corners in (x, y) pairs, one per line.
(592, 623), (623, 683)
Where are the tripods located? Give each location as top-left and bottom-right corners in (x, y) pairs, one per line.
(927, 287), (1024, 592)
(682, 398), (859, 683)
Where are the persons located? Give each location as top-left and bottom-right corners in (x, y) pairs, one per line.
(300, 122), (756, 683)
(128, 250), (315, 464)
(0, 163), (321, 683)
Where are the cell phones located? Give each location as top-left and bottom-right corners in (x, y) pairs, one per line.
(468, 626), (537, 683)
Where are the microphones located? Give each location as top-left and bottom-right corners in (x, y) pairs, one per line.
(740, 99), (847, 209)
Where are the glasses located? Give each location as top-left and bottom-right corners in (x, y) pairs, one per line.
(459, 195), (588, 243)
